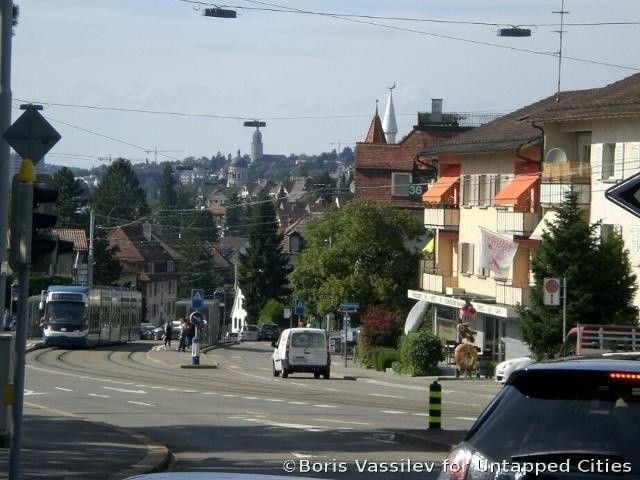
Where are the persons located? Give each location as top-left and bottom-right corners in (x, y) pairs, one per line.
(3, 309), (12, 330)
(175, 317), (195, 352)
(164, 320), (172, 349)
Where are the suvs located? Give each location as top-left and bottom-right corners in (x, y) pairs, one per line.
(260, 323), (282, 341)
(239, 322), (260, 342)
(437, 350), (639, 479)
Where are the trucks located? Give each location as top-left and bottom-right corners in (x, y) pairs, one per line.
(546, 321), (640, 355)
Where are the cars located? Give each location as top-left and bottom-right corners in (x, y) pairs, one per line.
(494, 352), (537, 384)
(142, 320), (182, 341)
(223, 331), (240, 343)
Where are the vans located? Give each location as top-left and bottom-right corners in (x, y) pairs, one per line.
(272, 326), (332, 379)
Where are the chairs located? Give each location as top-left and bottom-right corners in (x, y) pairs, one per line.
(445, 339), (493, 378)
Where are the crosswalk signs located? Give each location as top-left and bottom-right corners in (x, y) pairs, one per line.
(191, 289), (204, 308)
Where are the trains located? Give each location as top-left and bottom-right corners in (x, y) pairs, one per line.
(37, 283), (145, 350)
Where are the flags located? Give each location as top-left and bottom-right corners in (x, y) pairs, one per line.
(476, 229), (520, 280)
